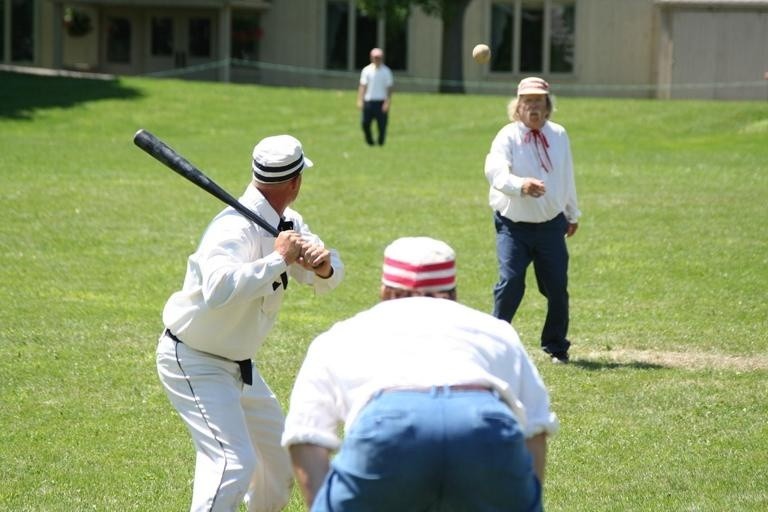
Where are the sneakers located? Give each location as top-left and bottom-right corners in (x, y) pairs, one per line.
(550, 351), (569, 365)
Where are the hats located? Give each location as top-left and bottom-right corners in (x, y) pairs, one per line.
(381, 237), (457, 292)
(516, 77), (550, 96)
(251, 135), (314, 184)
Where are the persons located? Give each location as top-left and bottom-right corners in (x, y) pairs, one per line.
(355, 47), (393, 147)
(281, 235), (555, 512)
(153, 133), (344, 512)
(483, 74), (580, 364)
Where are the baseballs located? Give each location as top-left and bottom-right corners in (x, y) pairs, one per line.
(473, 44), (491, 65)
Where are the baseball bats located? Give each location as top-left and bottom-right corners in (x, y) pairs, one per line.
(132, 130), (325, 269)
